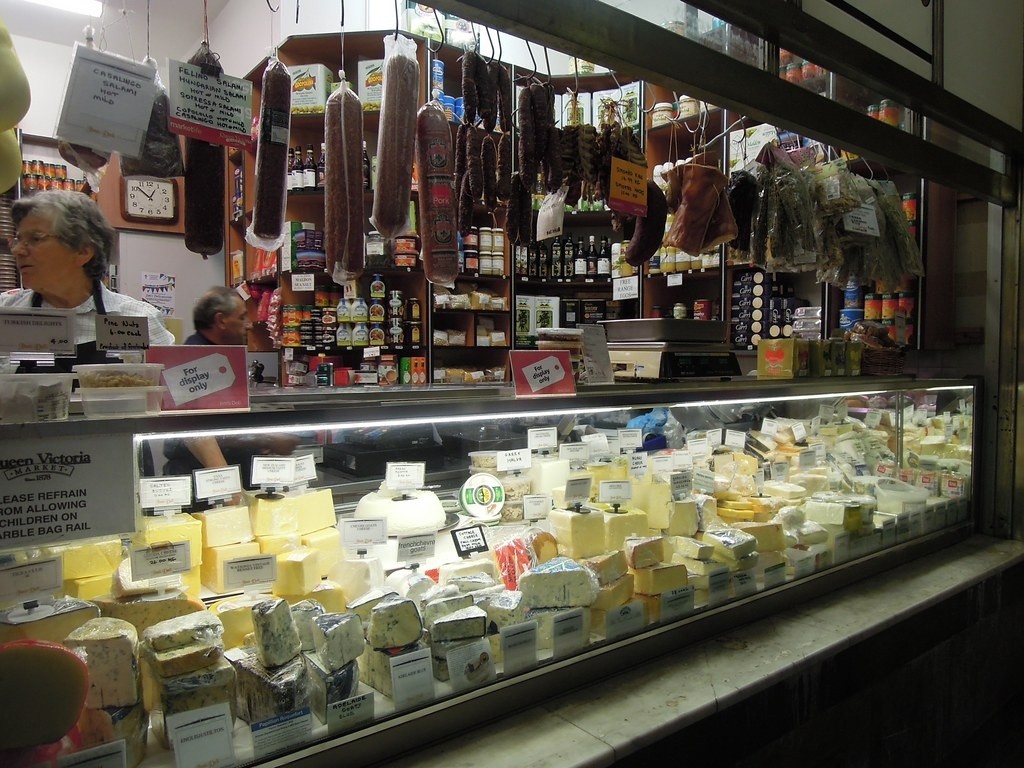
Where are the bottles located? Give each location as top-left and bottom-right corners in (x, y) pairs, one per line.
(362, 141), (378, 191)
(514, 234), (612, 278)
(287, 142), (326, 194)
(335, 271), (423, 346)
(365, 231), (390, 271)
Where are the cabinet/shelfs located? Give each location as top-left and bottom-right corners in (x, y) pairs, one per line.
(0, 129), (110, 294)
(222, 22), (956, 387)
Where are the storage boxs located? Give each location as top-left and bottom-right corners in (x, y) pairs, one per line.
(310, 357), (355, 386)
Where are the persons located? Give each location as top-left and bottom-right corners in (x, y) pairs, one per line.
(161, 284), (301, 516)
(1, 188), (242, 522)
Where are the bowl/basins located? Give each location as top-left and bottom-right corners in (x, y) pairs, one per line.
(0, 373), (72, 424)
(77, 386), (162, 419)
(72, 363), (164, 387)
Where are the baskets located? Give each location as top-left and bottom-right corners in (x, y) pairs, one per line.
(861, 346), (905, 377)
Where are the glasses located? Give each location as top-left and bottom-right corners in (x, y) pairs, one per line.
(8, 231), (76, 250)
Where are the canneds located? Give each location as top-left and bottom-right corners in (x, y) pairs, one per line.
(431, 59), (500, 131)
(652, 98), (716, 129)
(280, 231), (421, 349)
(22, 160), (96, 202)
(618, 213), (720, 277)
(692, 298), (713, 321)
(455, 225), (505, 276)
(776, 49), (919, 346)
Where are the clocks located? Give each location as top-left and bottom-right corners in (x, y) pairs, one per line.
(119, 174), (179, 224)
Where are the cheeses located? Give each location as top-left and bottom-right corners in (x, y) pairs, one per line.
(0, 420), (933, 768)
(864, 410), (971, 475)
